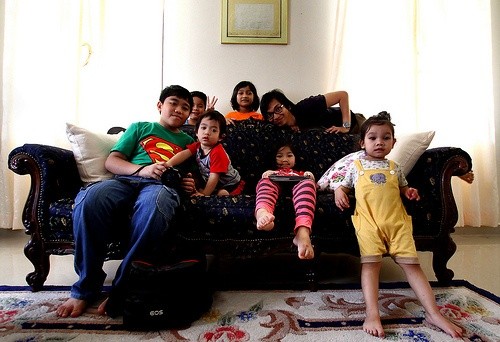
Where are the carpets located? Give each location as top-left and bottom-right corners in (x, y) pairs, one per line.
(1, 279), (500, 342)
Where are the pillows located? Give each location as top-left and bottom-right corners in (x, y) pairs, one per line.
(66, 123), (121, 184)
(317, 130), (436, 190)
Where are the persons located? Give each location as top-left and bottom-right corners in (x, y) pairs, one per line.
(182, 91), (218, 125)
(335, 111), (462, 338)
(223, 80), (262, 125)
(161, 111), (245, 197)
(56, 85), (195, 317)
(259, 89), (365, 134)
(255, 142), (317, 259)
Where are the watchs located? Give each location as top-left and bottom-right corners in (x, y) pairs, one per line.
(343, 122), (351, 128)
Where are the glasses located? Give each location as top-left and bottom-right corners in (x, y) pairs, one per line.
(264, 104), (284, 121)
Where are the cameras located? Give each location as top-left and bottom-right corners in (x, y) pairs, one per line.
(160, 166), (190, 188)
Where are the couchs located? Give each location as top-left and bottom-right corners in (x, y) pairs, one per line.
(8, 116), (475, 292)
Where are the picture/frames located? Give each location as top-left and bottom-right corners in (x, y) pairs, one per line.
(221, 0), (288, 45)
